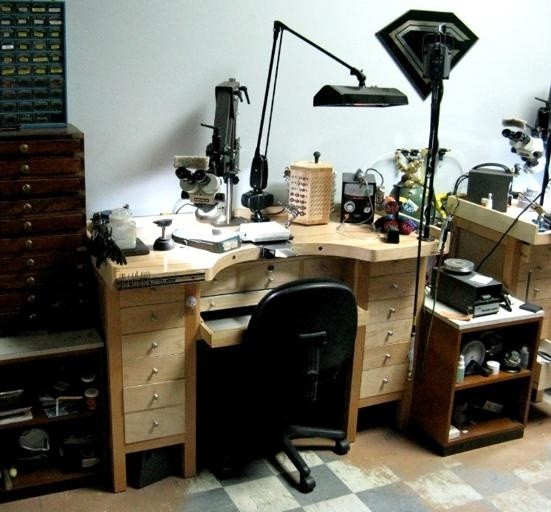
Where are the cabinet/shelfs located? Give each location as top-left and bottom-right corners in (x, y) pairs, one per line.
(404, 291), (547, 455)
(115, 279), (188, 448)
(194, 256), (370, 350)
(446, 192), (550, 416)
(359, 259), (420, 401)
(0, 0), (124, 504)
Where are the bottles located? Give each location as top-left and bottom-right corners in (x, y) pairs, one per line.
(79, 373), (99, 412)
(106, 207), (137, 250)
(456, 355), (466, 384)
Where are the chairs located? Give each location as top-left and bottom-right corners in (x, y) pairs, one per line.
(194, 274), (359, 492)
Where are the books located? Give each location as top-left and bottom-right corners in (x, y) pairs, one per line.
(0, 381), (43, 425)
